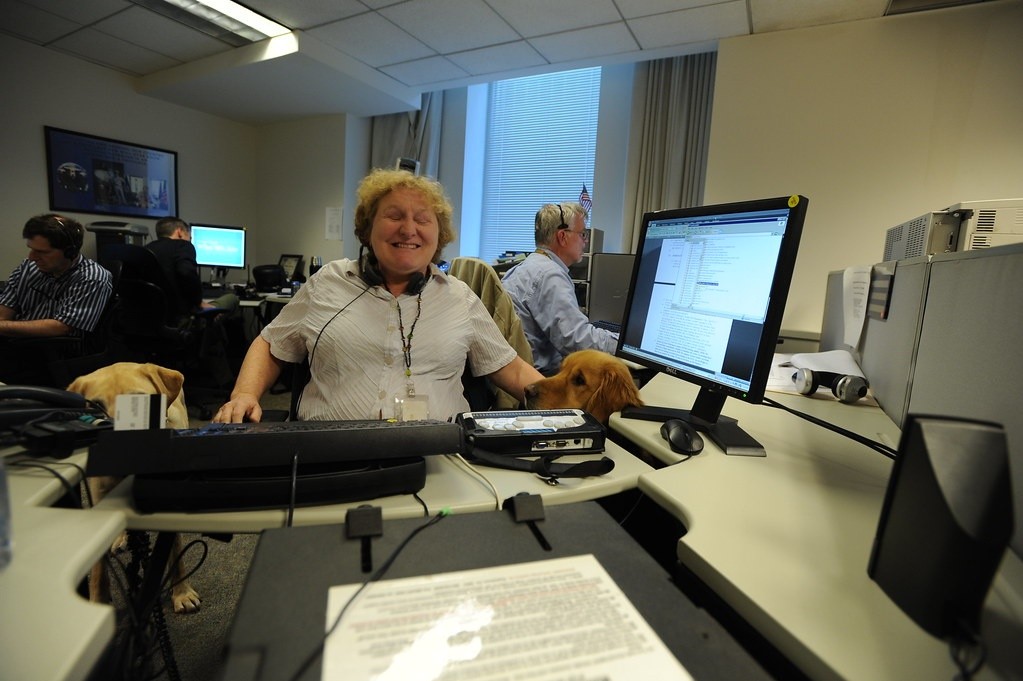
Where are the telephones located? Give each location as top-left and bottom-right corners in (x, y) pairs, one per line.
(0, 385), (104, 426)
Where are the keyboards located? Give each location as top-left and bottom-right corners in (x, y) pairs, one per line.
(590, 320), (621, 333)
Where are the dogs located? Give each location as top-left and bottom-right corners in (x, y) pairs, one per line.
(525, 347), (648, 432)
(64, 361), (204, 613)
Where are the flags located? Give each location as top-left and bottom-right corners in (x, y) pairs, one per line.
(579, 185), (592, 212)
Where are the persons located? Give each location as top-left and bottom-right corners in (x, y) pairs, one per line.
(78, 166), (152, 205)
(211, 170), (545, 424)
(0, 214), (113, 385)
(500, 203), (619, 378)
(143, 215), (240, 394)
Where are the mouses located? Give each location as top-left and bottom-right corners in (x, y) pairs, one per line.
(660, 418), (704, 456)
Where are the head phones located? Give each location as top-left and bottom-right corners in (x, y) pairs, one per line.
(358, 245), (431, 295)
(791, 367), (870, 403)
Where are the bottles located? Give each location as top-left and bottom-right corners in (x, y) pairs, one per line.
(291, 279), (300, 297)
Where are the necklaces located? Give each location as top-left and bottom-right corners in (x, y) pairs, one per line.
(378, 279), (422, 397)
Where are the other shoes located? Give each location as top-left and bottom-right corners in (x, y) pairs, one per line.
(195, 389), (232, 405)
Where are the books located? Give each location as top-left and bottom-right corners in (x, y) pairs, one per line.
(706, 422), (766, 456)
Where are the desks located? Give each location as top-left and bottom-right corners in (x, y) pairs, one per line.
(608, 370), (1023, 681)
(202, 283), (278, 391)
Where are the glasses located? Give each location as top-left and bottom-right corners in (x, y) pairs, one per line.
(561, 227), (589, 241)
(54, 216), (76, 251)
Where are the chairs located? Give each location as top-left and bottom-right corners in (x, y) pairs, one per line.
(0, 259), (124, 390)
(102, 244), (232, 420)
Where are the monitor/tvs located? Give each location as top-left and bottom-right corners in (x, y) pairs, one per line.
(615, 195), (809, 457)
(188, 223), (246, 270)
